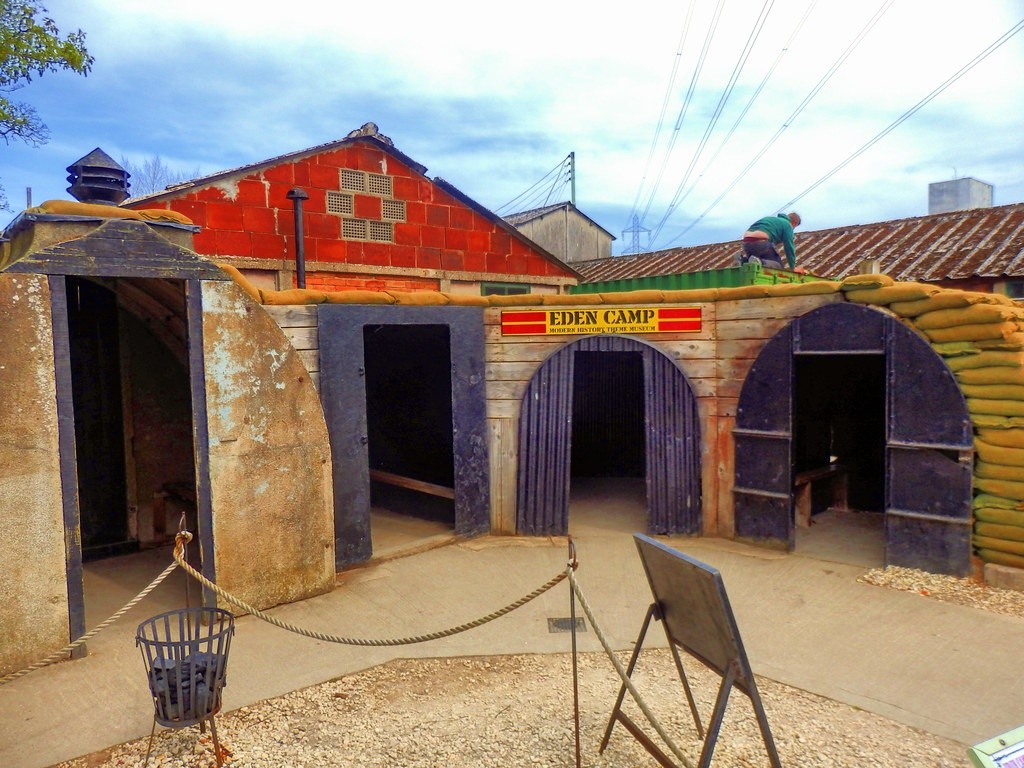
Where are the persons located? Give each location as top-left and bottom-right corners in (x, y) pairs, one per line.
(740, 212), (808, 276)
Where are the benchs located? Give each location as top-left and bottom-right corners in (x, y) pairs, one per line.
(794, 462), (850, 525)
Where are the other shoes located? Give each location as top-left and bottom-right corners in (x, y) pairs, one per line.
(748, 255), (761, 270)
(731, 252), (742, 267)
